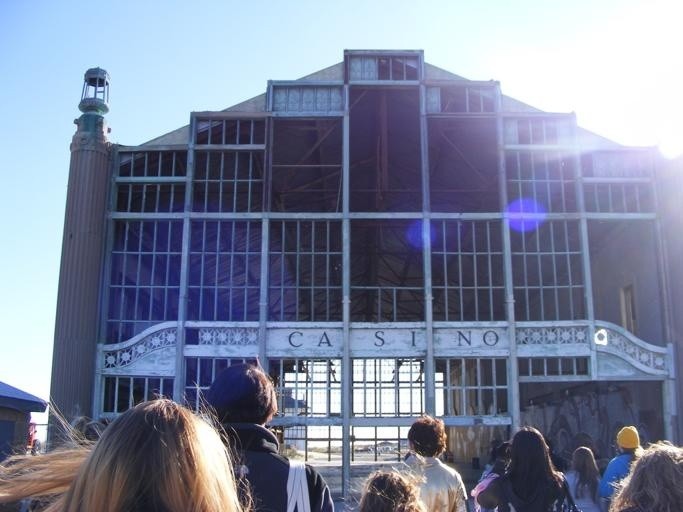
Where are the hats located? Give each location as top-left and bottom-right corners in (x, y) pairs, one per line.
(617, 425), (640, 449)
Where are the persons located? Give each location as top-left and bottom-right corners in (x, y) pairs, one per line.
(565, 448), (601, 512)
(18, 399), (252, 512)
(471, 427), (569, 511)
(599, 425), (639, 498)
(201, 361), (336, 511)
(613, 442), (683, 511)
(361, 473), (418, 512)
(399, 416), (467, 511)
